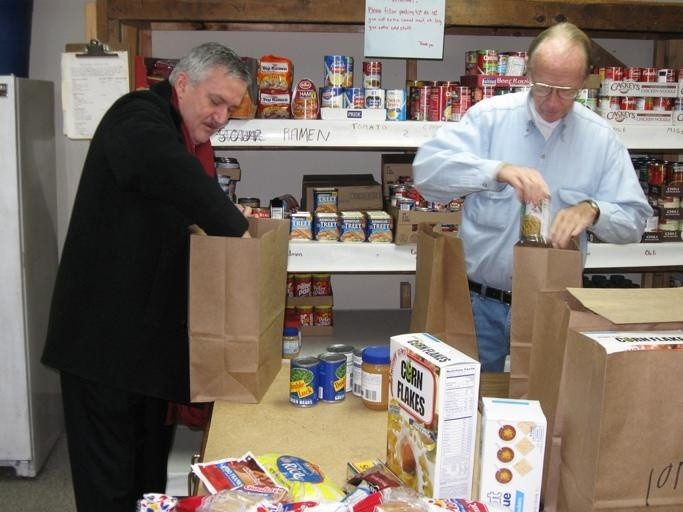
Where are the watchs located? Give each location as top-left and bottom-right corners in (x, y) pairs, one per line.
(582, 198), (599, 211)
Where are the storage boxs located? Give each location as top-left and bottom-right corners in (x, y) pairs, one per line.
(389, 330), (480, 502)
(554, 324), (681, 512)
(479, 393), (548, 512)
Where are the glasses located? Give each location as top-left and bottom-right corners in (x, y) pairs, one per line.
(528, 78), (579, 101)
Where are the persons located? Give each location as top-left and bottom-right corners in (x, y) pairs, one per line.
(40, 42), (252, 511)
(411, 22), (654, 371)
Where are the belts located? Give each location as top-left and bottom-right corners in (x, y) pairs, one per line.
(467, 279), (510, 303)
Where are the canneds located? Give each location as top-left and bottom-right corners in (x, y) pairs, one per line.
(347, 56), (354, 86)
(363, 61), (382, 89)
(319, 86), (343, 109)
(629, 156), (683, 241)
(499, 54), (510, 75)
(508, 50), (526, 75)
(297, 305), (315, 327)
(441, 82), (457, 118)
(323, 55), (347, 87)
(408, 85), (429, 122)
(293, 273), (312, 296)
(213, 156), (272, 218)
(314, 304), (332, 326)
(385, 89), (409, 120)
(292, 186), (393, 244)
(281, 328), (300, 358)
(481, 86), (496, 99)
(464, 50), (477, 75)
(357, 345), (392, 410)
(352, 347), (365, 397)
(285, 305), (295, 318)
(326, 345), (353, 393)
(365, 88), (384, 110)
(428, 86), (447, 121)
(392, 176), (462, 232)
(285, 273), (294, 297)
(343, 87), (364, 109)
(450, 86), (473, 121)
(310, 273), (331, 296)
(289, 356), (320, 407)
(571, 61), (683, 109)
(477, 49), (498, 76)
(320, 352), (347, 403)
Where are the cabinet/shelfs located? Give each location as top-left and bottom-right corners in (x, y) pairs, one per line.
(84, 1), (683, 363)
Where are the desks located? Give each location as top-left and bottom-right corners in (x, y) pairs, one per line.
(188, 357), (389, 495)
